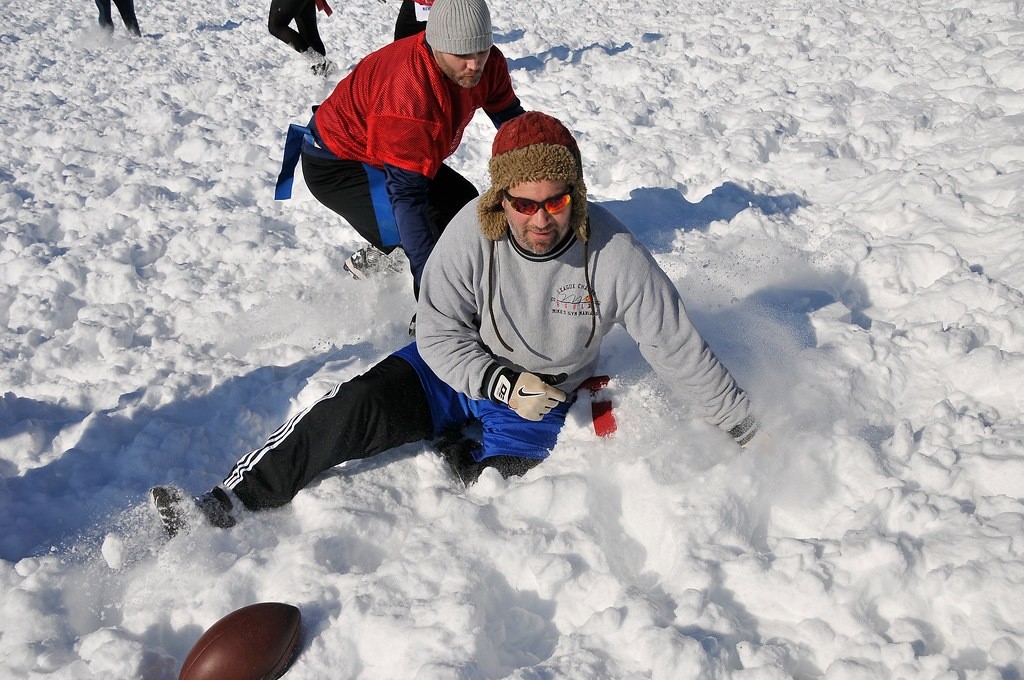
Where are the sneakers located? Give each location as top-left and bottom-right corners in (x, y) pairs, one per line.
(344, 247), (403, 283)
(150, 486), (237, 540)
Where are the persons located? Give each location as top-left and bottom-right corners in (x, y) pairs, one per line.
(268, 0), (335, 76)
(302, 0), (525, 337)
(151, 111), (761, 536)
(95, 0), (141, 36)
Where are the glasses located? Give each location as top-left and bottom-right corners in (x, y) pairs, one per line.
(501, 187), (572, 216)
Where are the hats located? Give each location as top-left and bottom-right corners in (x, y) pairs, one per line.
(425, 0), (494, 55)
(477, 111), (588, 245)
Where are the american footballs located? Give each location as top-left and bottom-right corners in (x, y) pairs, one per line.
(178, 602), (303, 680)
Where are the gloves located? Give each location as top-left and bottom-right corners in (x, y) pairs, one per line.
(480, 361), (572, 422)
(729, 412), (773, 453)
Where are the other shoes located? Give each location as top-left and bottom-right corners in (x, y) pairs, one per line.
(310, 58), (338, 79)
